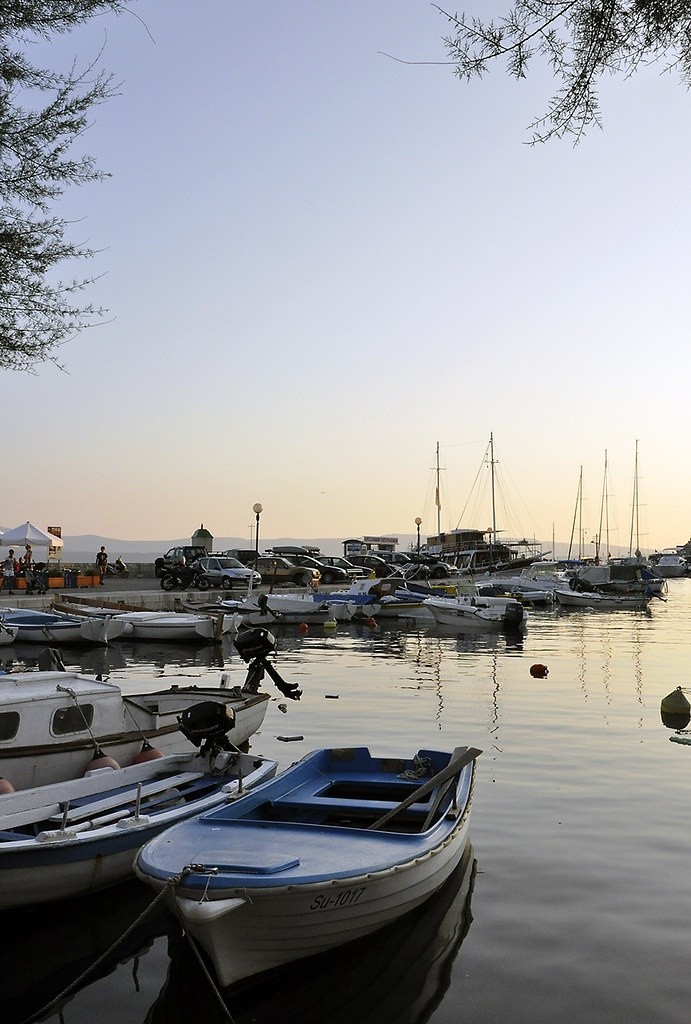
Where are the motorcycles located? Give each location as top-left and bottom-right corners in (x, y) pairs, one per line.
(161, 558), (211, 591)
(106, 555), (130, 579)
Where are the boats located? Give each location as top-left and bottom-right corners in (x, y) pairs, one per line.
(131, 746), (483, 997)
(49, 597), (244, 640)
(142, 837), (478, 1024)
(1, 751), (278, 914)
(1, 671), (272, 796)
(421, 594), (530, 626)
(0, 605), (128, 648)
(0, 641), (132, 676)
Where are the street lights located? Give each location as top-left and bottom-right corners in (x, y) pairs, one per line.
(253, 504), (264, 571)
(487, 528), (493, 565)
(414, 517), (423, 569)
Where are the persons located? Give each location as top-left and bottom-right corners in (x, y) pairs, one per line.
(95, 546), (109, 585)
(23, 544), (34, 571)
(182, 546), (194, 591)
(0, 549), (17, 595)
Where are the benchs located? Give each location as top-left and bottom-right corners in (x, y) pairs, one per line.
(275, 771), (438, 817)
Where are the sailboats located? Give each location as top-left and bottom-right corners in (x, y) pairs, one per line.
(173, 432), (691, 629)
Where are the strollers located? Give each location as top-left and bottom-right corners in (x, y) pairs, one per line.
(18, 565), (46, 595)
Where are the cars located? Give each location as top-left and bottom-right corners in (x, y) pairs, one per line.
(282, 556), (347, 586)
(186, 557), (263, 590)
(335, 554), (406, 577)
(399, 551), (459, 579)
(244, 556), (323, 587)
(313, 556), (376, 580)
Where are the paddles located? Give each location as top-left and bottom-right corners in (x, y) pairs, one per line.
(38, 778), (227, 843)
(367, 747), (484, 831)
(418, 747), (469, 835)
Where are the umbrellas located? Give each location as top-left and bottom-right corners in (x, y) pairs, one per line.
(0, 520), (65, 563)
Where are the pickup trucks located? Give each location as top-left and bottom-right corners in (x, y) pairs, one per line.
(154, 546), (326, 578)
(366, 549), (431, 580)
